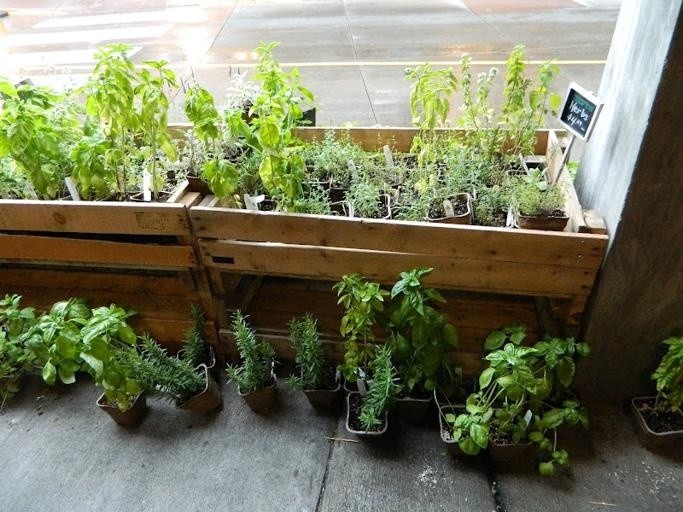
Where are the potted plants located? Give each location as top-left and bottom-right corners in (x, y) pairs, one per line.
(83, 338), (147, 426)
(284, 310), (344, 408)
(435, 324), (592, 476)
(225, 308), (282, 414)
(175, 302), (216, 378)
(632, 334), (682, 447)
(1, 43), (569, 230)
(332, 267), (459, 444)
(113, 336), (222, 413)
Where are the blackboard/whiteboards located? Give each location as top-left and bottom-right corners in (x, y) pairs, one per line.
(559, 86), (597, 138)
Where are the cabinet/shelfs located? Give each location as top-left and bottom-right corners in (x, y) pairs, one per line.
(0, 123), (218, 344)
(190, 125), (608, 378)
